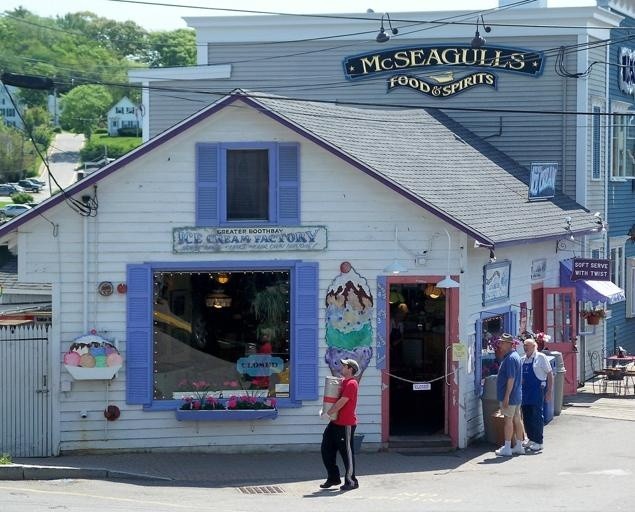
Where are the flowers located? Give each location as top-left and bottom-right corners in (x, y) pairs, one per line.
(580, 308), (609, 319)
(178, 379), (219, 410)
(225, 377), (276, 408)
(489, 336), (521, 351)
(518, 329), (551, 343)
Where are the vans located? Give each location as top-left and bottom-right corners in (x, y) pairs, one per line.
(152, 272), (289, 359)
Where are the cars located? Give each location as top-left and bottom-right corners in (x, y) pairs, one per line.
(0, 178), (47, 197)
(0, 203), (39, 219)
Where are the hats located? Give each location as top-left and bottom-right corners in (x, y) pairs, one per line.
(340, 358), (359, 372)
(498, 333), (513, 343)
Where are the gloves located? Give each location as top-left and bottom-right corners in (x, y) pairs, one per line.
(319, 409), (330, 421)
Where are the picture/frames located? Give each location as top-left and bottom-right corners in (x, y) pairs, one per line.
(481, 258), (512, 307)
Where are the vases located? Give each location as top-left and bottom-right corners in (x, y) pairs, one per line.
(587, 316), (601, 325)
(495, 348), (514, 359)
(537, 341), (545, 352)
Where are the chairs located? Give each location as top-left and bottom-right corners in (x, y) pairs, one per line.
(587, 343), (635, 398)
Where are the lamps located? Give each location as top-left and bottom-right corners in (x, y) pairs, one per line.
(472, 240), (497, 265)
(471, 13), (492, 48)
(217, 273), (229, 284)
(375, 12), (398, 43)
(563, 217), (575, 241)
(206, 285), (232, 309)
(383, 223), (460, 289)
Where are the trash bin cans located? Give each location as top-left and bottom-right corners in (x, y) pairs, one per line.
(480, 374), (514, 443)
(544, 355), (556, 425)
(540, 350), (567, 416)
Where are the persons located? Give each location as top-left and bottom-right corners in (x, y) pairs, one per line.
(494, 333), (525, 456)
(521, 338), (553, 451)
(319, 358), (359, 491)
(390, 310), (406, 384)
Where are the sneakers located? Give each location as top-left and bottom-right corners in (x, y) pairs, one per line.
(510, 445), (525, 455)
(522, 440), (534, 450)
(495, 445), (513, 456)
(319, 479), (341, 488)
(525, 443), (544, 452)
(340, 482), (358, 490)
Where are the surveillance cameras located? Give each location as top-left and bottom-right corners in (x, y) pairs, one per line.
(82, 196), (90, 204)
